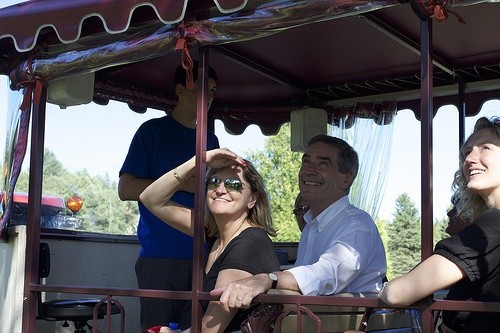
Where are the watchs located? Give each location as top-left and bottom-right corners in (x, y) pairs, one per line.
(265, 271), (278, 289)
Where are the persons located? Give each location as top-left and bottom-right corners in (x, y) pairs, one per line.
(118, 59), (221, 331)
(379, 115), (500, 333)
(138, 135), (387, 332)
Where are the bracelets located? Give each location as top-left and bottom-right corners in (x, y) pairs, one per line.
(173, 166), (185, 183)
(379, 281), (388, 293)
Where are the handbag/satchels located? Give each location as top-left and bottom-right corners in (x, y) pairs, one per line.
(240, 303), (284, 333)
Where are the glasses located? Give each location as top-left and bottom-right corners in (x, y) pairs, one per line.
(206, 176), (255, 192)
(293, 204), (309, 216)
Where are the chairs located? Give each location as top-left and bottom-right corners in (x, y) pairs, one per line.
(35, 241), (121, 333)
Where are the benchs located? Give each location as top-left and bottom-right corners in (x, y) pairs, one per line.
(240, 288), (422, 333)
